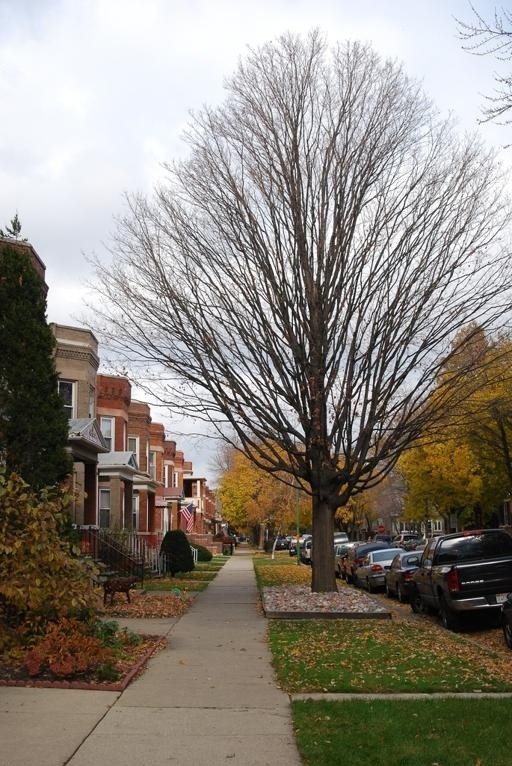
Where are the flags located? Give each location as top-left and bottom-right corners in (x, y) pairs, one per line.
(180, 502), (193, 534)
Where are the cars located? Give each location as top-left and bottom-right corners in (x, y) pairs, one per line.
(275, 528), (511, 649)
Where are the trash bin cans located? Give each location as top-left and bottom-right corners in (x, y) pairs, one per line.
(222, 536), (236, 556)
